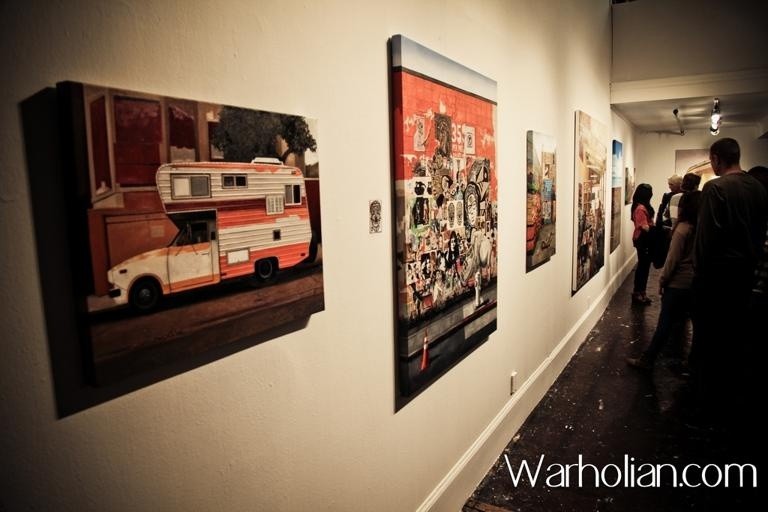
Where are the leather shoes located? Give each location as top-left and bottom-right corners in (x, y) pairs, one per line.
(625, 356), (653, 371)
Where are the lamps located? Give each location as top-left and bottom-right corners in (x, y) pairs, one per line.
(710, 99), (722, 136)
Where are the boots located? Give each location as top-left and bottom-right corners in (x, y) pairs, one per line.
(631, 291), (652, 305)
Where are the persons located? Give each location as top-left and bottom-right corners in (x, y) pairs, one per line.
(405, 116), (498, 312)
(623, 186), (710, 371)
(661, 173), (702, 234)
(672, 136), (768, 380)
(656, 176), (688, 224)
(629, 182), (656, 305)
(368, 199), (383, 234)
